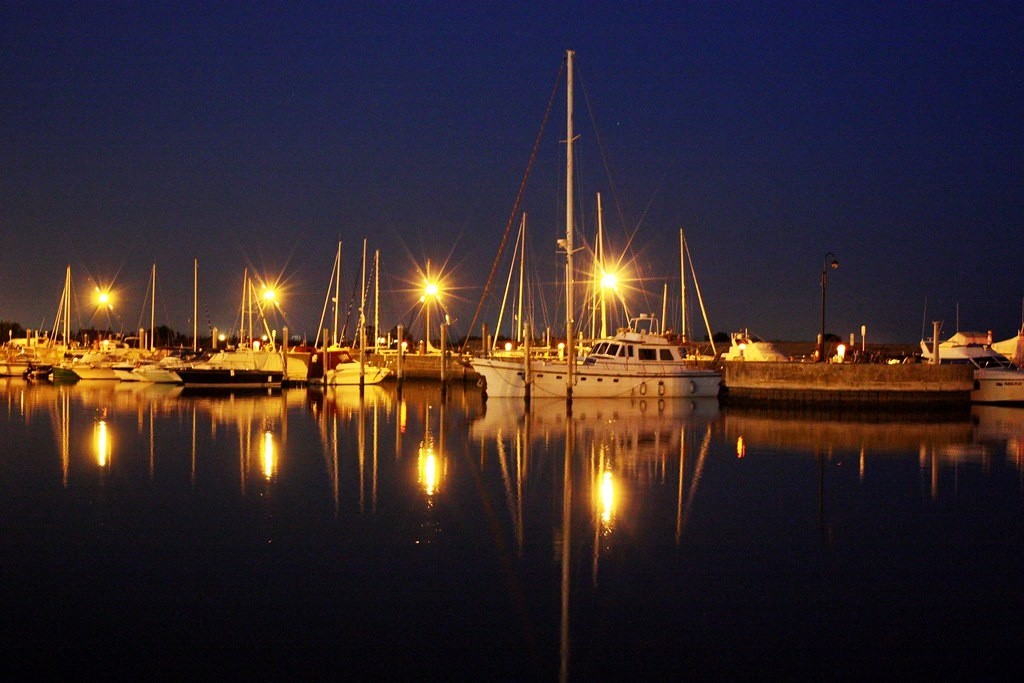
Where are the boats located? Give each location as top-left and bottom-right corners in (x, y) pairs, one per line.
(720, 327), (796, 364)
(0, 191), (716, 384)
(914, 294), (1024, 401)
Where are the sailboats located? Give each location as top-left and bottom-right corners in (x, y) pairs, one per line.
(448, 50), (724, 398)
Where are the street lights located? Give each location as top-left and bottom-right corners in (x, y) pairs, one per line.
(820, 255), (838, 358)
